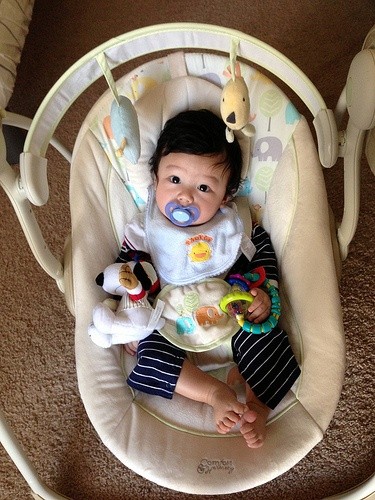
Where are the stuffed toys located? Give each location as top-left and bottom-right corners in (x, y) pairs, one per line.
(220, 76), (257, 143)
(109, 96), (141, 165)
(87, 258), (165, 348)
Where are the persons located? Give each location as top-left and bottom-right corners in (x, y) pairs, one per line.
(110, 110), (302, 448)
